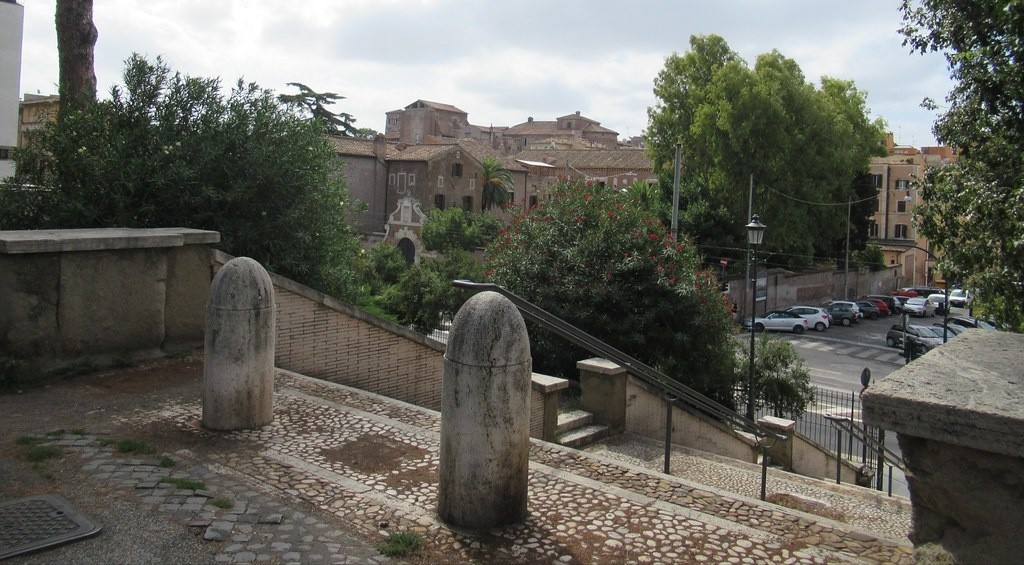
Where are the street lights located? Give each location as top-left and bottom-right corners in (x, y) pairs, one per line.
(899, 243), (950, 344)
(743, 213), (767, 423)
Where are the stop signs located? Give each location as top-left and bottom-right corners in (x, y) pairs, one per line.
(719, 258), (729, 267)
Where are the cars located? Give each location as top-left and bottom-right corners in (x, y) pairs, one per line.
(819, 285), (945, 327)
(886, 324), (944, 355)
(743, 310), (809, 334)
(901, 296), (935, 317)
(781, 305), (833, 332)
(933, 321), (967, 337)
(927, 293), (951, 315)
(972, 315), (1012, 331)
(944, 316), (997, 331)
(949, 288), (970, 308)
(924, 325), (956, 341)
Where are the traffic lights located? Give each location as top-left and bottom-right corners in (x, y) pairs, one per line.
(721, 280), (729, 292)
